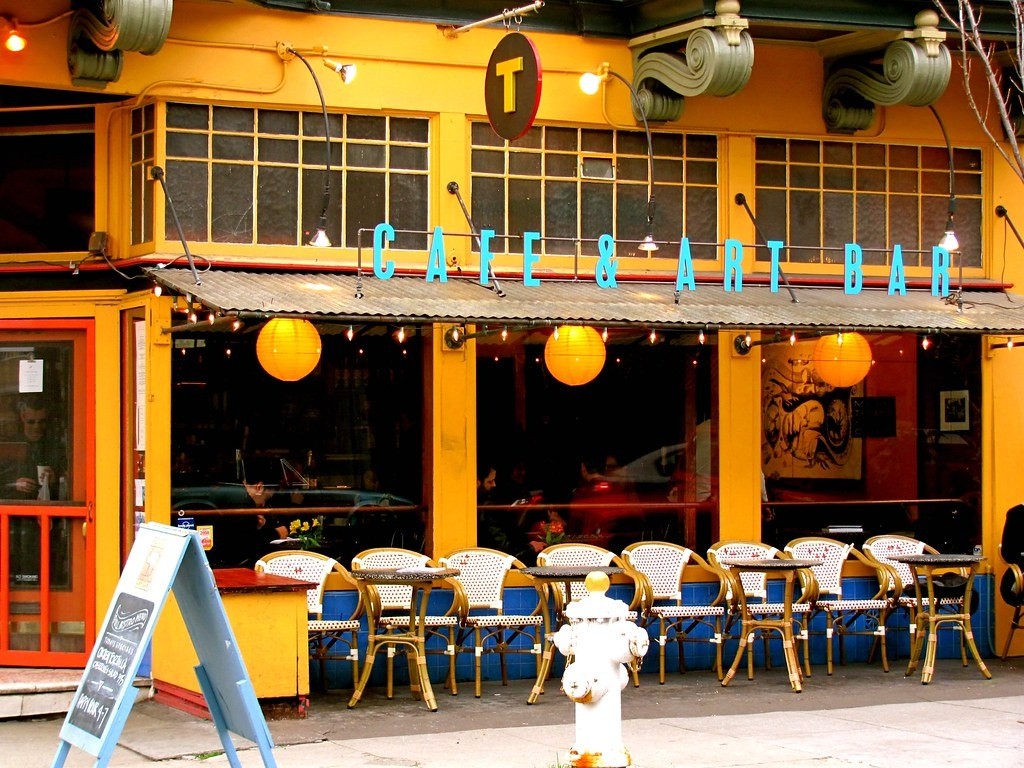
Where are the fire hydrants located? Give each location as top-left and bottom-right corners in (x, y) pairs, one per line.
(554, 570), (648, 767)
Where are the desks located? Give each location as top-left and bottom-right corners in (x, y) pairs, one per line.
(348, 567), (461, 714)
(716, 557), (825, 693)
(887, 552), (994, 684)
(517, 565), (627, 705)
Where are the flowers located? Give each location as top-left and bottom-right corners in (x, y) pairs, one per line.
(537, 521), (565, 546)
(288, 517), (322, 550)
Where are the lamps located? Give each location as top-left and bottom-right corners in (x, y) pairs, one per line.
(275, 37), (964, 254)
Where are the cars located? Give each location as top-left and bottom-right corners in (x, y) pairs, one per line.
(170, 457), (424, 566)
(519, 467), (920, 559)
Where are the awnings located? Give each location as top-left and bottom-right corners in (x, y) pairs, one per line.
(144, 266), (1024, 334)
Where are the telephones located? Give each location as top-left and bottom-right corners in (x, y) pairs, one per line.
(56, 521), (192, 758)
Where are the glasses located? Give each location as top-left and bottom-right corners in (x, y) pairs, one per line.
(21, 412), (48, 424)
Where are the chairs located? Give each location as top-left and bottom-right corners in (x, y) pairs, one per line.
(253, 534), (968, 711)
(997, 543), (1023, 660)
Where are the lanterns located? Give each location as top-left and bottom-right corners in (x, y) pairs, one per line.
(257, 318), (323, 382)
(814, 333), (872, 387)
(544, 325), (607, 386)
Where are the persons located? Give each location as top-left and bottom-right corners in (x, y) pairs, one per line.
(0, 394), (68, 587)
(571, 448), (640, 535)
(207, 471), (276, 564)
(478, 463), (497, 548)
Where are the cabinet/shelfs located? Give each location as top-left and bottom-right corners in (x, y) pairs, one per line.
(150, 569), (319, 722)
